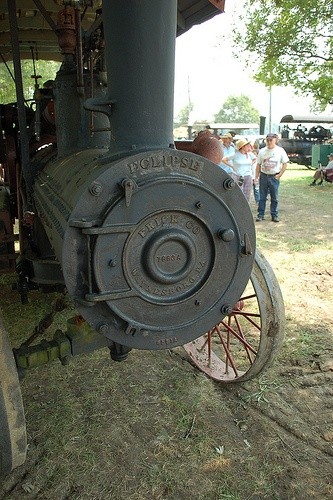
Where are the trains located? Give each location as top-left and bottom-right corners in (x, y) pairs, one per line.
(1, 0), (333, 476)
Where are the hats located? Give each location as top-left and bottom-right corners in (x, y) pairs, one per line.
(327, 152), (333, 158)
(236, 138), (251, 150)
(220, 133), (233, 139)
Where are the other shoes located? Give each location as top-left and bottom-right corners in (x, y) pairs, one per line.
(308, 182), (316, 186)
(271, 216), (279, 222)
(256, 216), (263, 221)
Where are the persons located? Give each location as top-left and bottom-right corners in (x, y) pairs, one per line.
(205, 125), (236, 178)
(222, 139), (269, 204)
(308, 153), (333, 186)
(254, 134), (289, 222)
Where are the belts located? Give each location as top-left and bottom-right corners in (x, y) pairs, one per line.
(261, 172), (279, 176)
